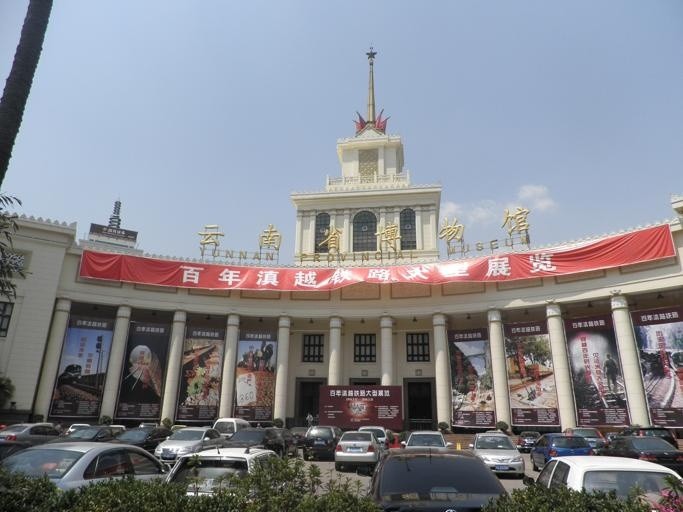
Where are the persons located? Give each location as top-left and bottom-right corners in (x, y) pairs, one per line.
(385, 431), (400, 448)
(603, 354), (618, 391)
(305, 412), (313, 427)
